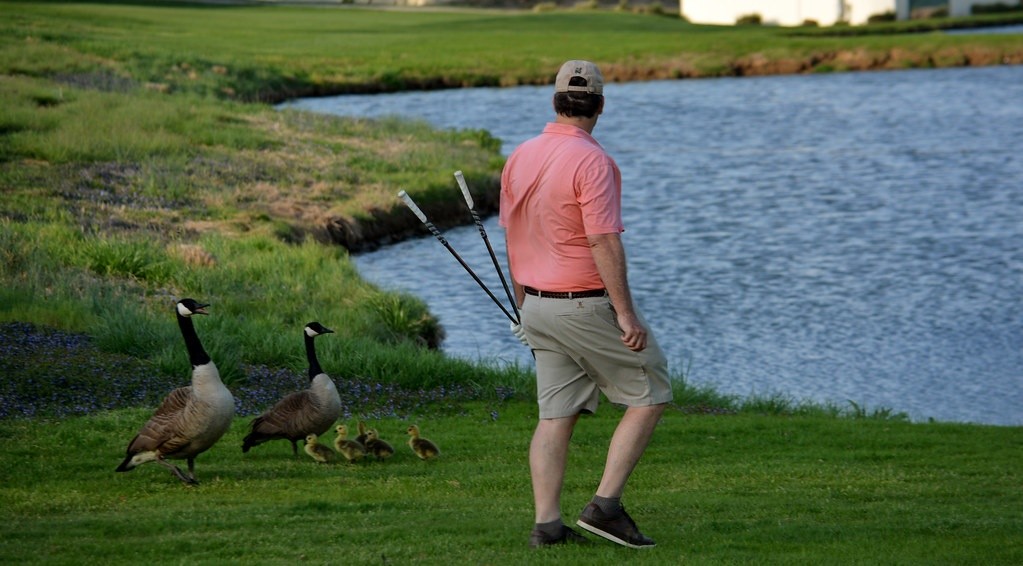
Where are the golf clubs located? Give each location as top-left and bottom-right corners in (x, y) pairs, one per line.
(396, 189), (520, 328)
(455, 170), (536, 362)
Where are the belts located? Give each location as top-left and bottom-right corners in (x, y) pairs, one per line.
(523, 285), (609, 299)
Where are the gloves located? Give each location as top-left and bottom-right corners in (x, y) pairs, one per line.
(510, 308), (528, 346)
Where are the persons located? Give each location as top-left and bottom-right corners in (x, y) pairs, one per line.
(499, 59), (673, 548)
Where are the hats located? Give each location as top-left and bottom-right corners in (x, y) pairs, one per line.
(554, 59), (604, 96)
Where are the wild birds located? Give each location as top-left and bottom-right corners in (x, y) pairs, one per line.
(241, 321), (343, 460)
(115, 299), (235, 485)
(304, 421), (394, 463)
(404, 424), (440, 460)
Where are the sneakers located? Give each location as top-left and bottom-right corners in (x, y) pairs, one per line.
(529, 524), (591, 548)
(576, 504), (657, 550)
